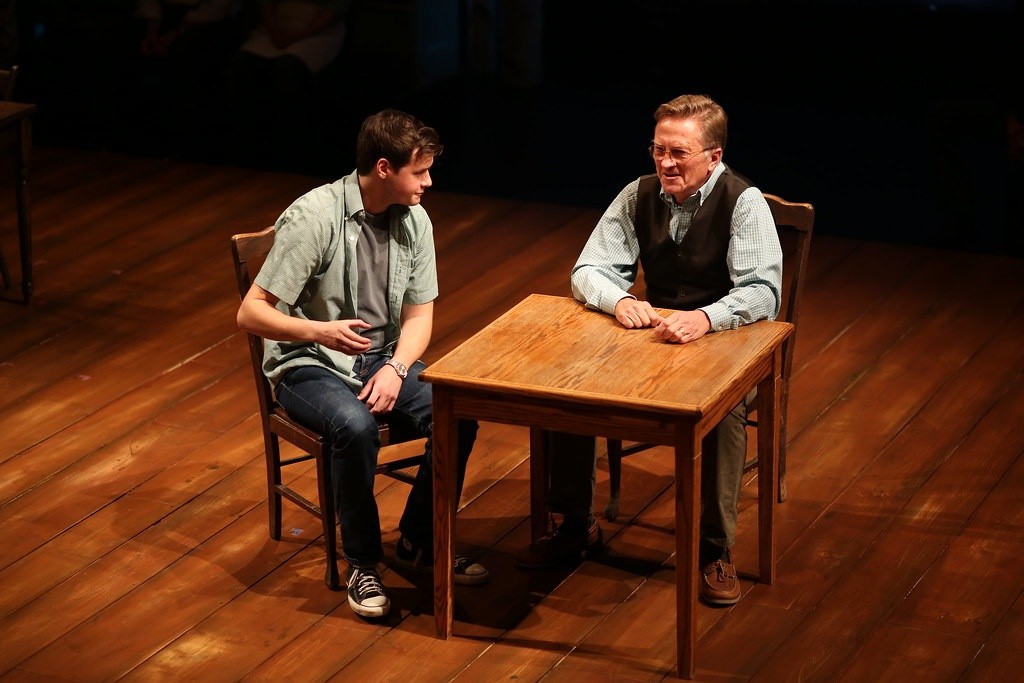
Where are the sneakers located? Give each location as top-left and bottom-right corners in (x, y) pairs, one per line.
(395, 535), (491, 584)
(346, 564), (391, 618)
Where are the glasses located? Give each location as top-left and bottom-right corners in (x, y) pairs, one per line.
(646, 145), (717, 163)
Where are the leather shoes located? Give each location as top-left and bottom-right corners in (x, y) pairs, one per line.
(529, 518), (603, 555)
(701, 547), (741, 605)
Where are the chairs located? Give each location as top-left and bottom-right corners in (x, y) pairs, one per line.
(604, 192), (814, 521)
(232, 227), (427, 589)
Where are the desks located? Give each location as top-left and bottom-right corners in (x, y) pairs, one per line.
(419, 293), (794, 679)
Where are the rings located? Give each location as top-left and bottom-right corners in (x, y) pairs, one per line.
(678, 328), (686, 337)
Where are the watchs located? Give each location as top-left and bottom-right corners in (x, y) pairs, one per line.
(384, 359), (409, 380)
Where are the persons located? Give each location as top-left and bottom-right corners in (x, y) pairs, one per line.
(512, 92), (785, 608)
(235, 110), (494, 620)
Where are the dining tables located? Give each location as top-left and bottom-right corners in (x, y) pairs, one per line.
(0, 100), (42, 304)
